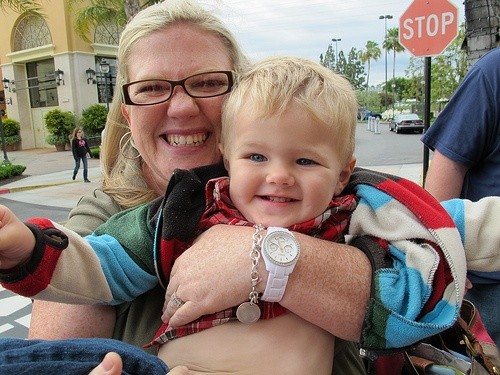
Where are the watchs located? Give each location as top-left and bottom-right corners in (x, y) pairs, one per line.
(260, 227), (300, 303)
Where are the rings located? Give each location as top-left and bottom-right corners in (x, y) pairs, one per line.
(169, 293), (183, 308)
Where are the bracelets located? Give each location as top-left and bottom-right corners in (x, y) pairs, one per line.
(236, 224), (265, 325)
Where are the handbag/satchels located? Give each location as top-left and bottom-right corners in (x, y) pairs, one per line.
(374, 299), (500, 375)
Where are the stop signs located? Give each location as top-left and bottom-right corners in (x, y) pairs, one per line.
(399, 0), (459, 57)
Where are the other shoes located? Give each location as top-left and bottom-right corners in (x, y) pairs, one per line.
(84, 179), (91, 183)
(73, 175), (76, 180)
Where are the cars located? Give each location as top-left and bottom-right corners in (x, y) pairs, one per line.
(359, 108), (382, 120)
(389, 114), (424, 133)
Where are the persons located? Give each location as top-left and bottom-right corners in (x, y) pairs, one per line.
(72, 128), (94, 183)
(420, 46), (500, 353)
(0, 53), (500, 375)
(29, 0), (500, 375)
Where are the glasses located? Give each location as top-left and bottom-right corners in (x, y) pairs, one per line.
(122, 71), (236, 106)
(77, 132), (82, 133)
